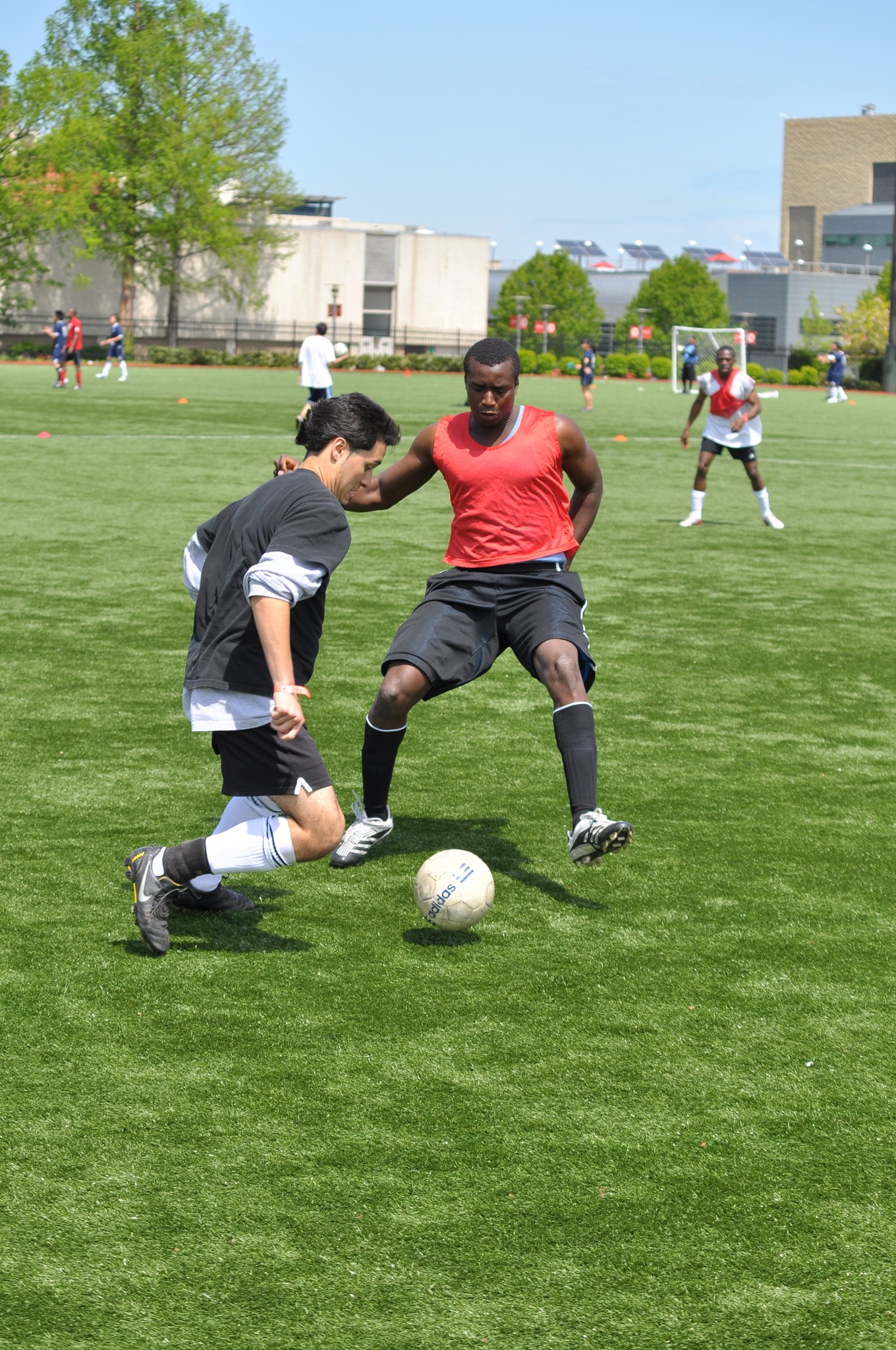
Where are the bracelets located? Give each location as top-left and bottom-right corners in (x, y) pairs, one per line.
(273, 685), (311, 699)
(742, 413), (748, 421)
(63, 345), (66, 347)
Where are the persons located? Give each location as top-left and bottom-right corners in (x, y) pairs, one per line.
(124, 392), (399, 956)
(95, 313), (128, 381)
(575, 338), (595, 412)
(815, 341), (848, 403)
(679, 345), (784, 529)
(55, 307), (82, 389)
(273, 340), (634, 865)
(296, 322), (349, 428)
(373, 361), (387, 371)
(40, 309), (68, 387)
(465, 400), (469, 406)
(678, 336), (697, 394)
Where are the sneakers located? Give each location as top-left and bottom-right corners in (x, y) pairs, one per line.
(125, 845), (182, 955)
(330, 790), (394, 867)
(567, 807), (635, 868)
(173, 881), (254, 913)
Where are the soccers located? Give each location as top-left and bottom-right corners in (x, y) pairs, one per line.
(412, 848), (496, 935)
(677, 344), (684, 352)
(333, 342), (348, 357)
(827, 353), (836, 361)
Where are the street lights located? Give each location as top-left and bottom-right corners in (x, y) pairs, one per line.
(329, 283), (344, 344)
(538, 304), (558, 354)
(509, 295), (533, 350)
(633, 308), (653, 354)
(603, 319), (616, 354)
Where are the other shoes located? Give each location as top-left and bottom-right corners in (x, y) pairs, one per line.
(296, 415), (304, 429)
(826, 397), (837, 403)
(838, 394), (848, 402)
(763, 510), (784, 529)
(680, 510), (702, 526)
